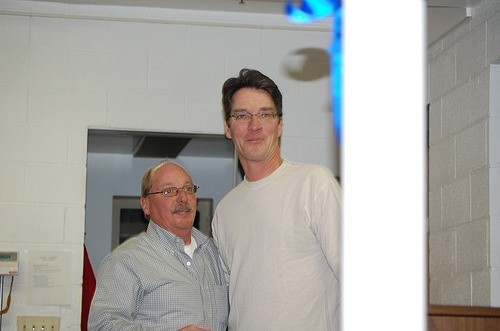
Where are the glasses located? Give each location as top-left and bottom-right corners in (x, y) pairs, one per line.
(227, 112), (279, 123)
(146, 185), (199, 197)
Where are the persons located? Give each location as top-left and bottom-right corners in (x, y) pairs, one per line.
(87, 162), (229, 331)
(211, 70), (343, 331)
